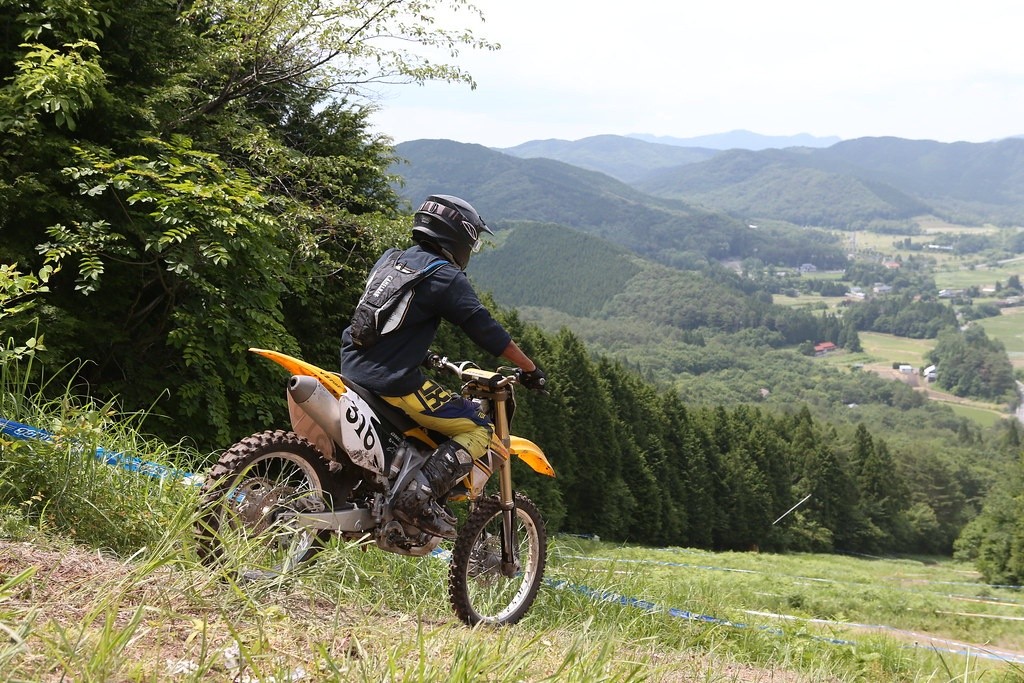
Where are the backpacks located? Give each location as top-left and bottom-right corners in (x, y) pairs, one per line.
(350, 251), (451, 348)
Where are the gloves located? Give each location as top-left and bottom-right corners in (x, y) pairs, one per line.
(519, 365), (545, 391)
(421, 350), (433, 370)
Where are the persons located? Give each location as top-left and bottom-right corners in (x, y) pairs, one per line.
(340, 195), (547, 539)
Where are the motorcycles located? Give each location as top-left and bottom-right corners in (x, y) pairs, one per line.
(196, 346), (554, 628)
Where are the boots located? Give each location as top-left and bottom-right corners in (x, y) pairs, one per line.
(393, 440), (474, 538)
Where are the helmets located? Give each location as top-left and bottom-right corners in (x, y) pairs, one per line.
(412, 194), (494, 270)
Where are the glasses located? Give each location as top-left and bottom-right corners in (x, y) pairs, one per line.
(472, 239), (483, 253)
